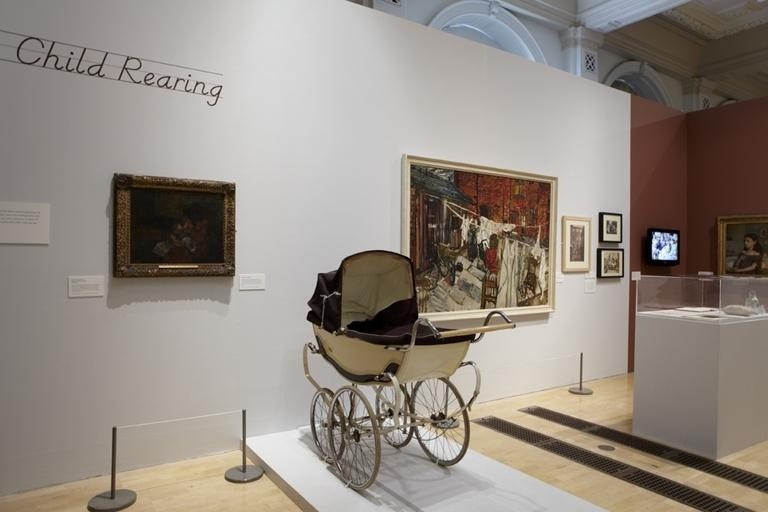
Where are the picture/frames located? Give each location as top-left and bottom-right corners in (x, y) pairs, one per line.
(398, 151), (560, 321)
(597, 248), (625, 279)
(109, 166), (240, 281)
(562, 214), (593, 274)
(597, 211), (624, 244)
(715, 213), (767, 276)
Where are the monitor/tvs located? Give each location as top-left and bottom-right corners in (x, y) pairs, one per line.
(646, 227), (680, 266)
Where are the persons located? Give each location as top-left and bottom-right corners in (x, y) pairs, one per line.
(171, 216), (198, 256)
(150, 203), (212, 264)
(732, 233), (761, 274)
(466, 217), (481, 262)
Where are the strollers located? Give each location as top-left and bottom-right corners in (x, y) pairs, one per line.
(301, 248), (515, 494)
